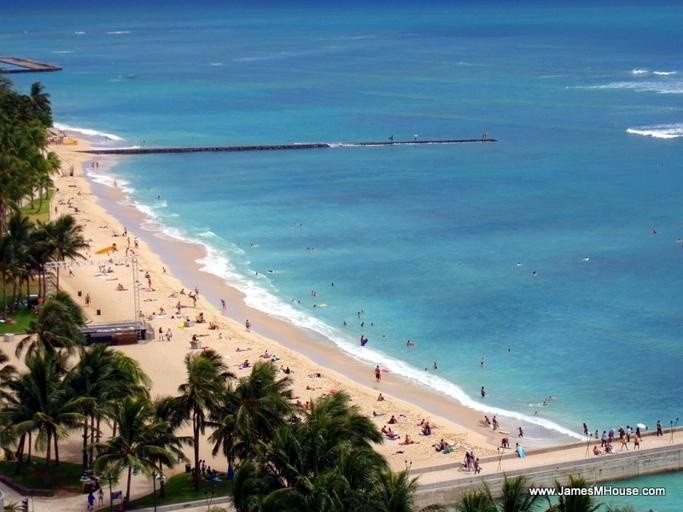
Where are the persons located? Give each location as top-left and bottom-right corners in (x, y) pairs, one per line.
(580, 417), (667, 456)
(1, 155), (557, 512)
